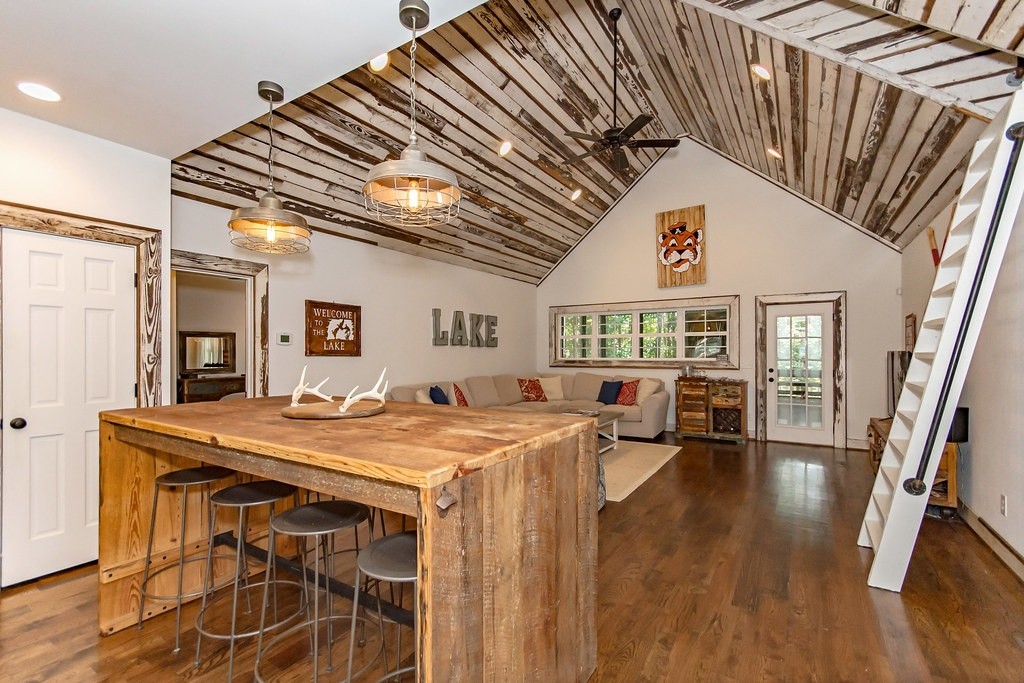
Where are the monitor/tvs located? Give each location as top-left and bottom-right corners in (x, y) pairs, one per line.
(878, 351), (912, 421)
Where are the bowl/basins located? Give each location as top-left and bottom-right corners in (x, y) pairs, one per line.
(180, 373), (198, 379)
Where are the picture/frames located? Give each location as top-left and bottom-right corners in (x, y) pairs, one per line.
(305, 300), (361, 357)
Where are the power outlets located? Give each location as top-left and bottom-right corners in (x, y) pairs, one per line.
(1000, 494), (1006, 517)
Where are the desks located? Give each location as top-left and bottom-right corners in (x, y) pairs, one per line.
(177, 376), (245, 403)
(99, 395), (597, 683)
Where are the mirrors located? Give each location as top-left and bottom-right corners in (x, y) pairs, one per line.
(179, 330), (236, 373)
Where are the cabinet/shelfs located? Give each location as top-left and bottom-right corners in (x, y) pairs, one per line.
(869, 418), (958, 509)
(675, 379), (748, 445)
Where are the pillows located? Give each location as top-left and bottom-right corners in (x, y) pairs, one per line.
(596, 381), (623, 405)
(534, 376), (564, 400)
(636, 377), (660, 406)
(616, 380), (639, 406)
(415, 389), (434, 404)
(517, 379), (548, 402)
(430, 385), (449, 405)
(447, 383), (469, 407)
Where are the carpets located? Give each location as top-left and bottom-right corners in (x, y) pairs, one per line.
(599, 440), (682, 503)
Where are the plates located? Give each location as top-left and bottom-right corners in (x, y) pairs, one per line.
(678, 376), (706, 382)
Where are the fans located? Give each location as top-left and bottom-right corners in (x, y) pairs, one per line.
(562, 8), (680, 170)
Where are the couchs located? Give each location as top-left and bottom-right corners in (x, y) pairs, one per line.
(384, 373), (670, 438)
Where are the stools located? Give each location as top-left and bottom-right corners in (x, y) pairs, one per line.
(137, 466), (417, 683)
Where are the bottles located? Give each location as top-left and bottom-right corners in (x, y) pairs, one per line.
(683, 364), (705, 378)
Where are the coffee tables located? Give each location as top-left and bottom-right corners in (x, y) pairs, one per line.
(546, 409), (624, 454)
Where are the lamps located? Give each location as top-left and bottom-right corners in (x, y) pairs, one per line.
(362, 0), (461, 226)
(228, 81), (312, 254)
